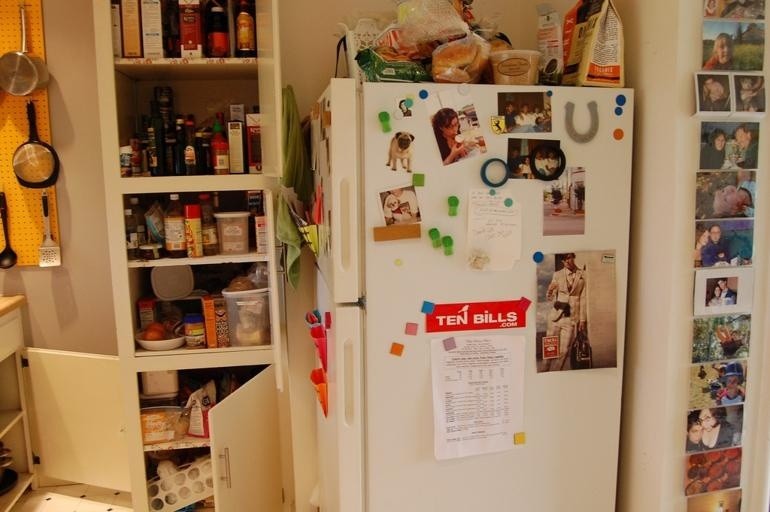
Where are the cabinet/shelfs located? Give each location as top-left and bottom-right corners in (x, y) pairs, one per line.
(90, 0), (303, 512)
(0, 291), (130, 512)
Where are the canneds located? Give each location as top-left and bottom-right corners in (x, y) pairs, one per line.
(184, 312), (206, 347)
(195, 132), (211, 173)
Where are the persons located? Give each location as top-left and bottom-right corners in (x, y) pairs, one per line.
(684, 0), (765, 452)
(504, 100), (551, 133)
(509, 148), (560, 179)
(383, 189), (419, 225)
(538, 253), (586, 371)
(433, 108), (468, 166)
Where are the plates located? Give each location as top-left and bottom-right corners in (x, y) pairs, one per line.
(135, 331), (187, 352)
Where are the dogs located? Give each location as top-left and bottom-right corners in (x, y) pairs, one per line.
(386, 131), (415, 172)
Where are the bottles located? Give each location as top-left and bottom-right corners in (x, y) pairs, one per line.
(206, 0), (256, 57)
(120, 82), (229, 177)
(123, 189), (252, 261)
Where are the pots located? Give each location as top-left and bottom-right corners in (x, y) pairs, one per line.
(0, 5), (50, 99)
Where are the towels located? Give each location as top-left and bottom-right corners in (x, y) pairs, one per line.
(278, 75), (315, 286)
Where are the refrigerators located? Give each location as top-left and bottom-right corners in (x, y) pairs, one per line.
(301, 75), (635, 512)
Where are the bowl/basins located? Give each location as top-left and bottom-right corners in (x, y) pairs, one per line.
(487, 50), (544, 85)
